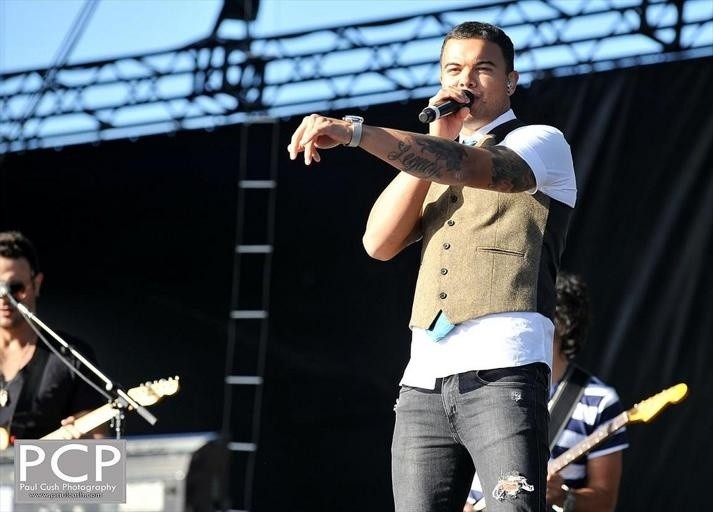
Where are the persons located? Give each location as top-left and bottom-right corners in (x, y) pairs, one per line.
(0, 230), (114, 439)
(459, 269), (631, 511)
(286, 20), (578, 512)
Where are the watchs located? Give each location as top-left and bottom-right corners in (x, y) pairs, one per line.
(562, 490), (576, 511)
(341, 114), (365, 148)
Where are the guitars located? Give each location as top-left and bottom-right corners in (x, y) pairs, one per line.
(36, 376), (182, 440)
(471, 386), (690, 511)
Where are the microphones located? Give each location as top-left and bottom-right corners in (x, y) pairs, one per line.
(419, 89), (475, 124)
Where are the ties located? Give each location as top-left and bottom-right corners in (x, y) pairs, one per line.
(461, 138), (478, 151)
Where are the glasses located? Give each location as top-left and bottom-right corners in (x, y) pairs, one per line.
(0, 273), (38, 301)
